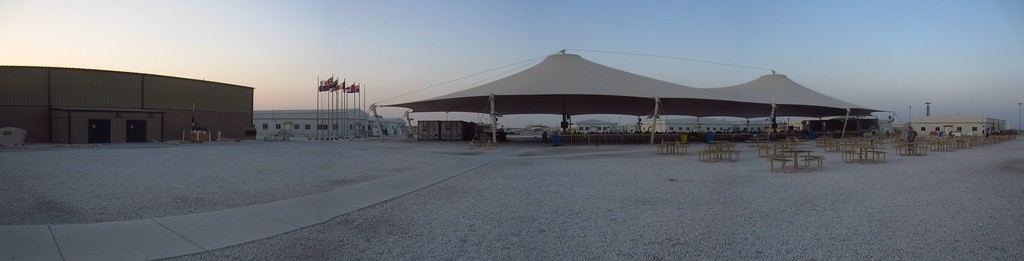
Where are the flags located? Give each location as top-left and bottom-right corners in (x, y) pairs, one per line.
(318, 78), (359, 93)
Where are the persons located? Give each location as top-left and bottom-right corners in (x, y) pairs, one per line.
(906, 126), (917, 151)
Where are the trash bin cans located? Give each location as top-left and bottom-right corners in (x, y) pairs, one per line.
(552, 134), (561, 146)
(705, 132), (715, 143)
(680, 132), (688, 144)
(811, 132), (816, 139)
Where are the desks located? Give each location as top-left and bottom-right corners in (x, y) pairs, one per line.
(779, 151), (814, 173)
(663, 142), (680, 155)
(708, 141), (734, 162)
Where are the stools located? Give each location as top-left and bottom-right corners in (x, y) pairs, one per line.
(745, 132), (890, 174)
(891, 135), (1013, 156)
(699, 141), (742, 163)
(657, 141), (690, 156)
